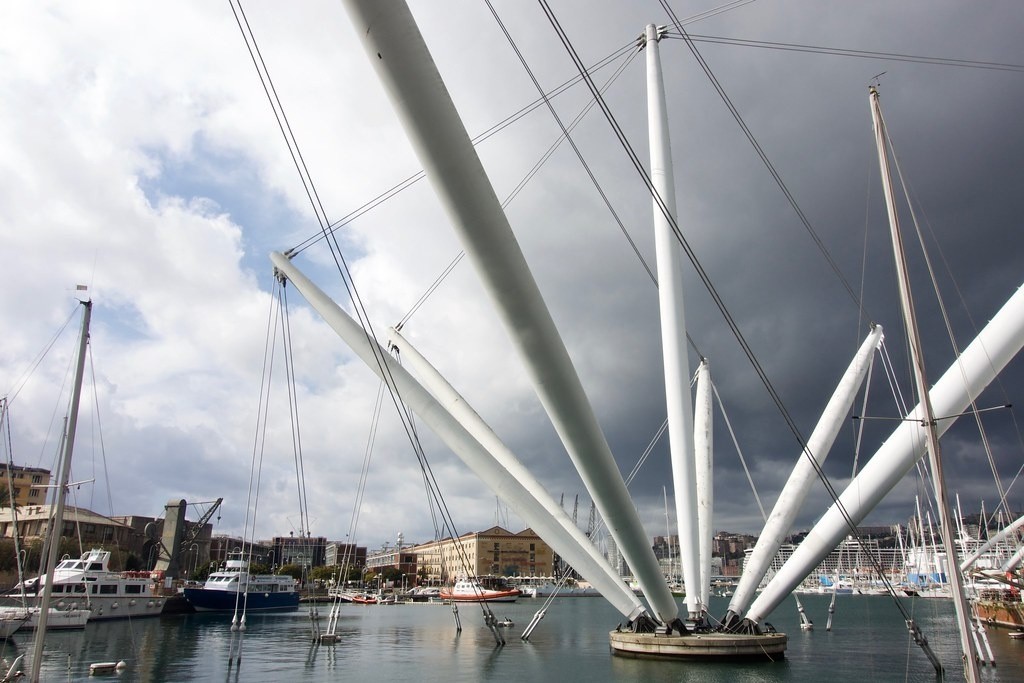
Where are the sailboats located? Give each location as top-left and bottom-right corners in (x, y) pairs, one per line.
(818, 494), (1024, 628)
(0, 397), (33, 638)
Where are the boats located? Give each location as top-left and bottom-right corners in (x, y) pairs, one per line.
(0, 551), (180, 619)
(353, 594), (377, 603)
(439, 581), (519, 602)
(184, 560), (299, 612)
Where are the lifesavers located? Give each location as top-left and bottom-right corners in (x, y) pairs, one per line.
(252, 575), (256, 581)
(1006, 571), (1016, 596)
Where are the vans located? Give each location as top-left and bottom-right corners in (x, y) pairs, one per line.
(149, 580), (184, 594)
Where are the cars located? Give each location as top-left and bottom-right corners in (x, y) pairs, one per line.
(676, 580), (684, 587)
(416, 588), (439, 595)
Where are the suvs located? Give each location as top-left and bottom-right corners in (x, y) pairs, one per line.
(710, 579), (732, 587)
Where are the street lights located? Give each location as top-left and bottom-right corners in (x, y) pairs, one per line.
(297, 552), (304, 589)
(267, 550), (275, 575)
(402, 574), (405, 594)
(210, 561), (218, 572)
(189, 543), (199, 571)
(348, 565), (353, 579)
(232, 547), (240, 560)
